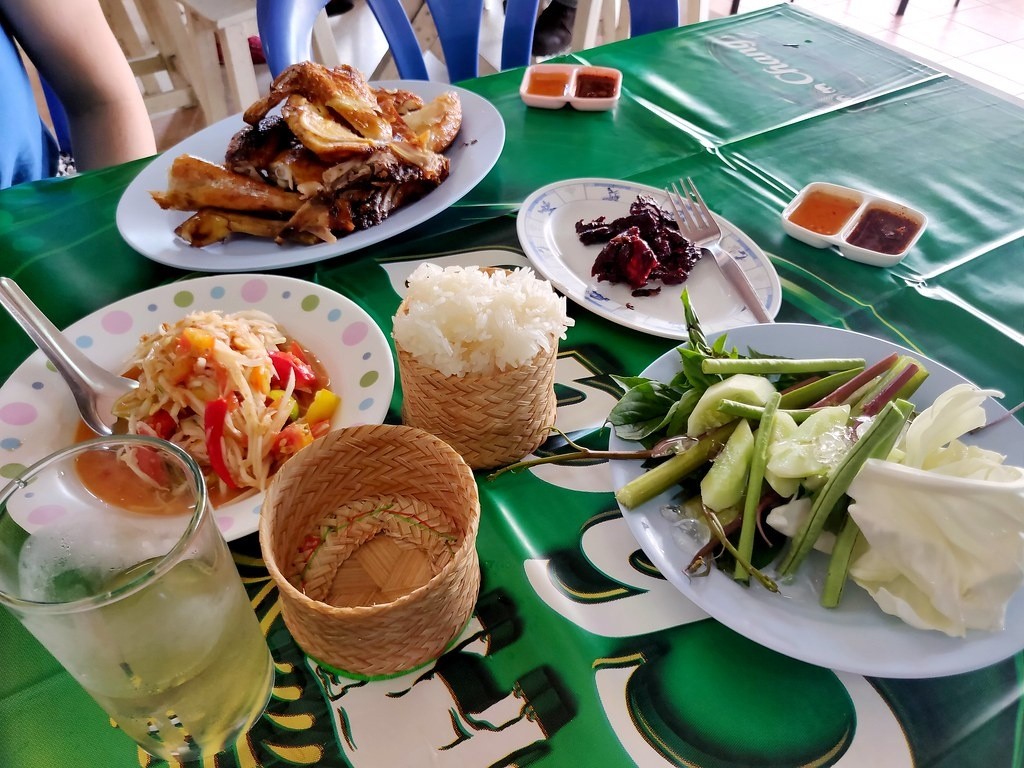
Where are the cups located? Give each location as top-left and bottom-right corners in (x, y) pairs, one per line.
(1, 434), (277, 762)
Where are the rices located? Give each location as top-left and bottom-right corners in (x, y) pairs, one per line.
(391, 262), (577, 376)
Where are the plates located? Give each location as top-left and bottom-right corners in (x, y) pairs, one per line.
(520, 63), (623, 111)
(115, 79), (506, 271)
(610, 321), (1023, 679)
(516, 177), (782, 340)
(780, 181), (929, 268)
(0, 275), (395, 542)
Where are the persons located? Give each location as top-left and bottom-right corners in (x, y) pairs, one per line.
(0, 0), (163, 187)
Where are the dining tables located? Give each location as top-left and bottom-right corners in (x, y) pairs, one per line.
(2, 0), (1023, 767)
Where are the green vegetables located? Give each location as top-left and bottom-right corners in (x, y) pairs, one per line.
(607, 292), (833, 445)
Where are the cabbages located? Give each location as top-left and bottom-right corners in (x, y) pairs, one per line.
(767, 386), (1022, 639)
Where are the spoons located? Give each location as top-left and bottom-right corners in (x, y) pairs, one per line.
(0, 271), (141, 436)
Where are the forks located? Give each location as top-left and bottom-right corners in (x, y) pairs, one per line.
(665, 177), (771, 325)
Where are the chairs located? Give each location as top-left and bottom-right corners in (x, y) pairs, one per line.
(257, 1), (679, 84)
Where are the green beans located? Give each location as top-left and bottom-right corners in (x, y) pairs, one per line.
(704, 357), (915, 609)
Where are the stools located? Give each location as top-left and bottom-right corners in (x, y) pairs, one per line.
(181, 0), (341, 125)
